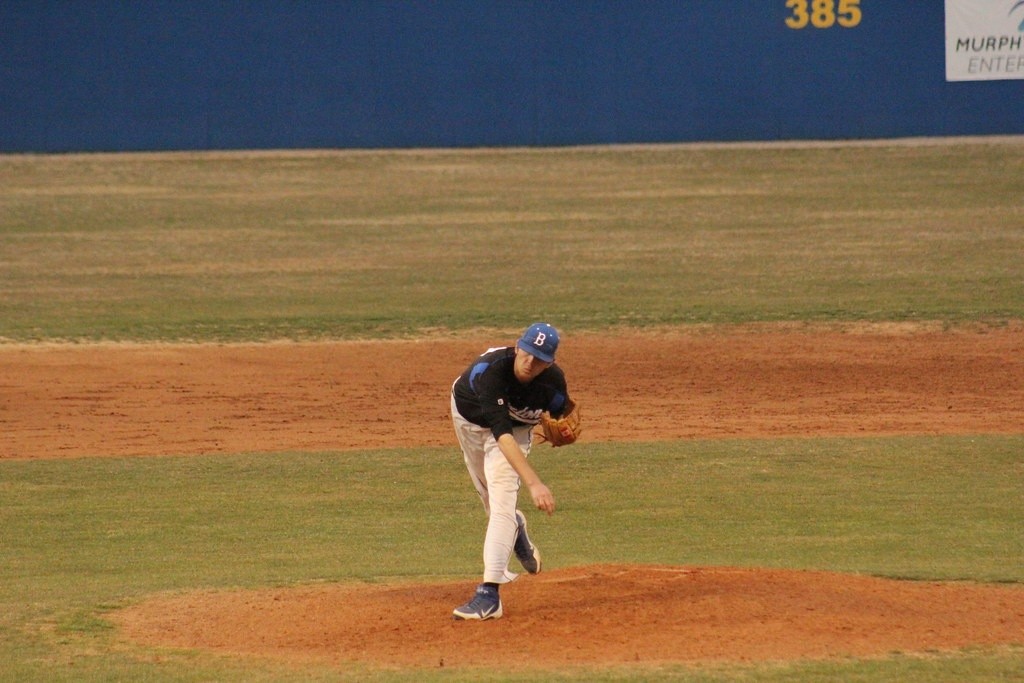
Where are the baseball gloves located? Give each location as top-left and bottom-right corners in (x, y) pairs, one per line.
(537, 398), (582, 448)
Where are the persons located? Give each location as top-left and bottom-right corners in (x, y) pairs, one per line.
(451, 323), (582, 621)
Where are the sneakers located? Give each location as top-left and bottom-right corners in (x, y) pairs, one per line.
(453, 584), (502, 620)
(514, 509), (541, 575)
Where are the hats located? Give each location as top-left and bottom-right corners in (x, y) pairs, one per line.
(517, 322), (560, 362)
